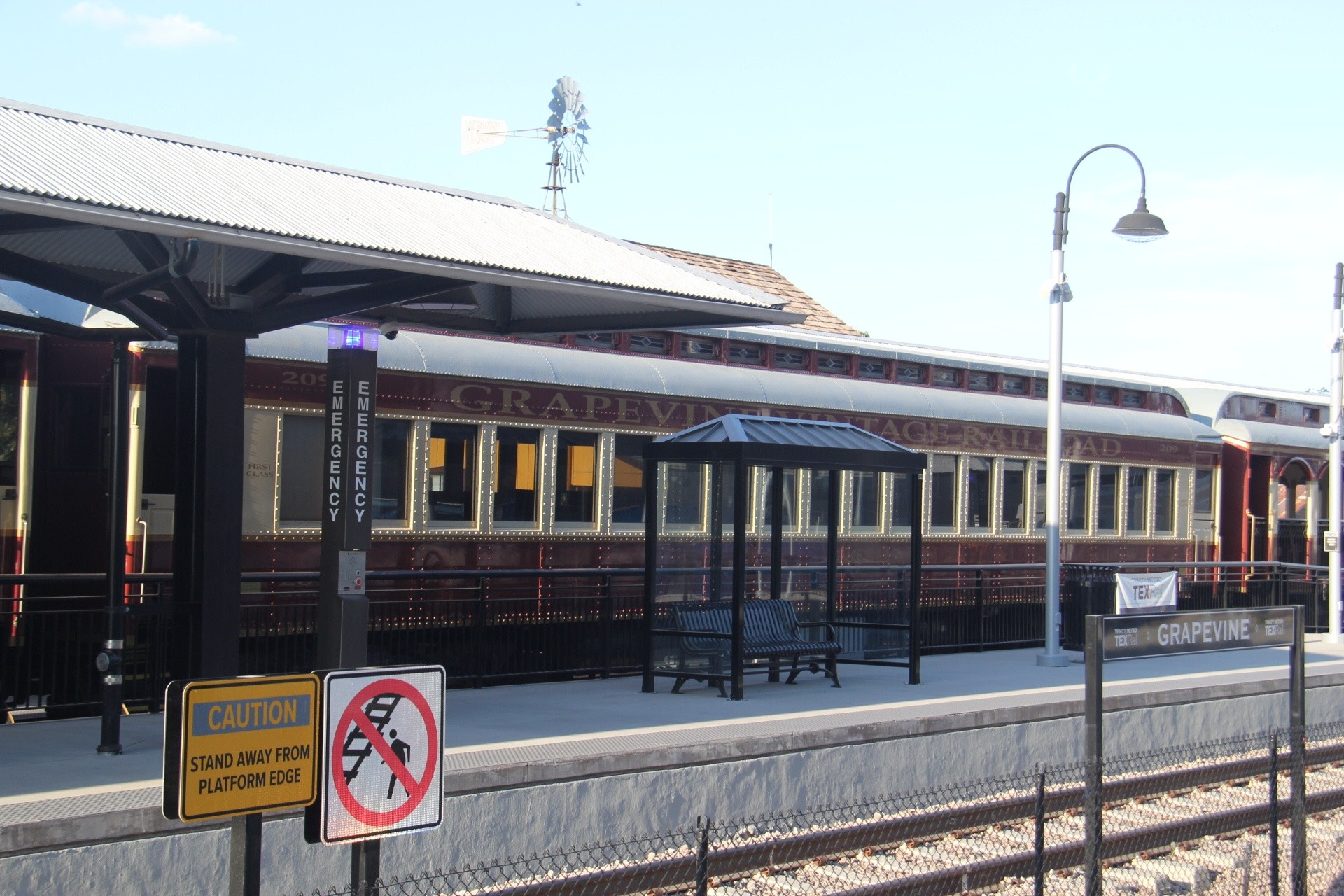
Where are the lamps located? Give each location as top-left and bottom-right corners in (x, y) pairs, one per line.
(1062, 143), (1170, 245)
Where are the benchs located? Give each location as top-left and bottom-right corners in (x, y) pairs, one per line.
(369, 482), (648, 524)
(667, 598), (843, 698)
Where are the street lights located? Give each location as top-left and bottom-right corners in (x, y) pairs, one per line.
(1035, 144), (1168, 669)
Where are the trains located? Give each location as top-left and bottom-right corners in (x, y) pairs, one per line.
(0, 280), (1329, 717)
